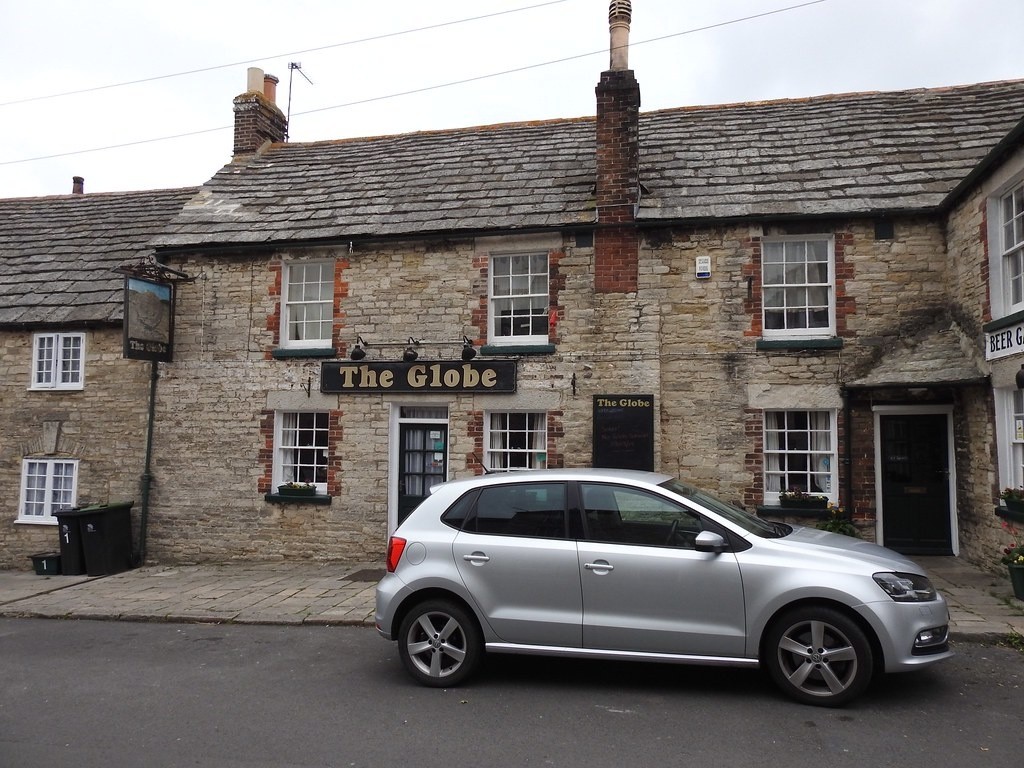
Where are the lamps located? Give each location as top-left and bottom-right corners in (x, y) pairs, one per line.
(461, 334), (477, 359)
(403, 337), (421, 360)
(350, 335), (369, 359)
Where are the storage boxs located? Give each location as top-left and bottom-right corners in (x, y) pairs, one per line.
(27, 552), (60, 576)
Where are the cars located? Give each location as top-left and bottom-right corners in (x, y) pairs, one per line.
(373, 467), (957, 708)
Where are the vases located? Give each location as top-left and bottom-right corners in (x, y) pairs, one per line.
(779, 496), (829, 508)
(1007, 563), (1024, 601)
(1005, 500), (1024, 512)
(277, 485), (316, 496)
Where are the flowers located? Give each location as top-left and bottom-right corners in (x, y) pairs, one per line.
(995, 485), (1024, 500)
(778, 486), (827, 502)
(285, 479), (313, 488)
(816, 500), (863, 537)
(999, 521), (1024, 565)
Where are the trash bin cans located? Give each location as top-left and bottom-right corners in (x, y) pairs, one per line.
(50, 507), (86, 575)
(73, 500), (135, 577)
